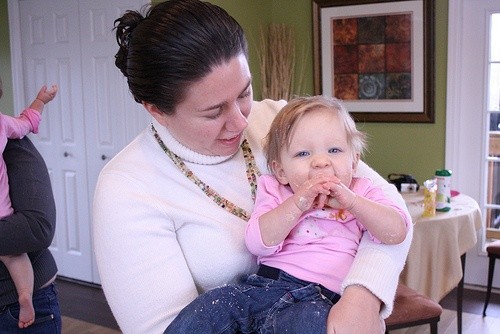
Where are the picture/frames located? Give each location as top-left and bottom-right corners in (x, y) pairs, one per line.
(311, 0), (435, 124)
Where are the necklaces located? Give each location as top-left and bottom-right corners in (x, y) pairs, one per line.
(152, 122), (262, 222)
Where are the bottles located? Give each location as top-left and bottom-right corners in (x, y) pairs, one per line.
(434, 168), (450, 210)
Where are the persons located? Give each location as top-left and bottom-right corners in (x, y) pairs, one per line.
(93, 0), (413, 334)
(0, 78), (57, 329)
(163, 95), (410, 334)
(0, 135), (63, 334)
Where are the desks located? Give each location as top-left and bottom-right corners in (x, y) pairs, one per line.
(397, 185), (483, 334)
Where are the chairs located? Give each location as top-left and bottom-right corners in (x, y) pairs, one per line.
(482, 240), (500, 318)
(384, 280), (442, 334)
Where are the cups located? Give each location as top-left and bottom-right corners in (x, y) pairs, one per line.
(424, 188), (437, 216)
